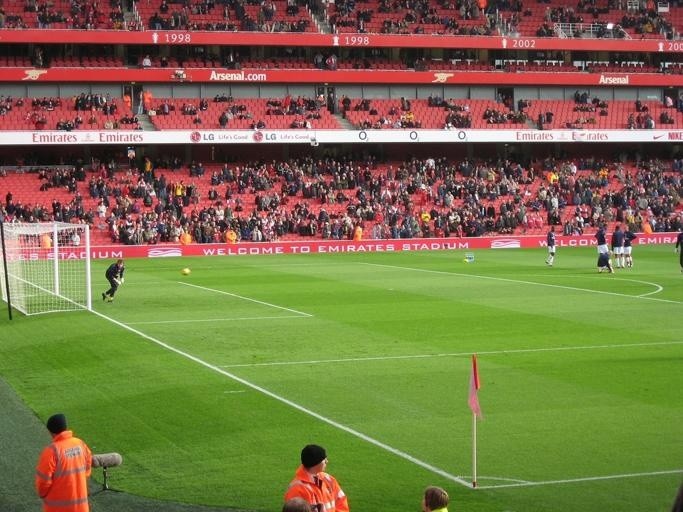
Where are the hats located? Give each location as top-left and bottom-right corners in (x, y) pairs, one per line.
(46, 413), (66, 433)
(302, 444), (326, 467)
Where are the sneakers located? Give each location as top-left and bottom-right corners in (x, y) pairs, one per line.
(103, 292), (115, 302)
(544, 260), (553, 267)
(598, 259), (633, 275)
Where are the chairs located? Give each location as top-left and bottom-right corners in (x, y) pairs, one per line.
(0, 154), (683, 245)
(0, 0), (683, 42)
(1, 81), (683, 133)
(0, 41), (683, 76)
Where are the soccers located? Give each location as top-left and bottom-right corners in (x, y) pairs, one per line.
(182, 268), (190, 276)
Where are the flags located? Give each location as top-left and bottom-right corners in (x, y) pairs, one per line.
(468, 354), (485, 423)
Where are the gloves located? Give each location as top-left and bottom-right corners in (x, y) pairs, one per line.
(113, 279), (124, 286)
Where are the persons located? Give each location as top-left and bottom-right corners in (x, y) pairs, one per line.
(674, 228), (683, 272)
(99, 259), (124, 302)
(543, 226), (556, 265)
(282, 495), (313, 511)
(594, 224), (608, 254)
(422, 486), (449, 512)
(35, 413), (92, 512)
(597, 251), (614, 273)
(611, 225), (625, 268)
(623, 226), (637, 267)
(284, 443), (348, 511)
(0, 1), (683, 247)
